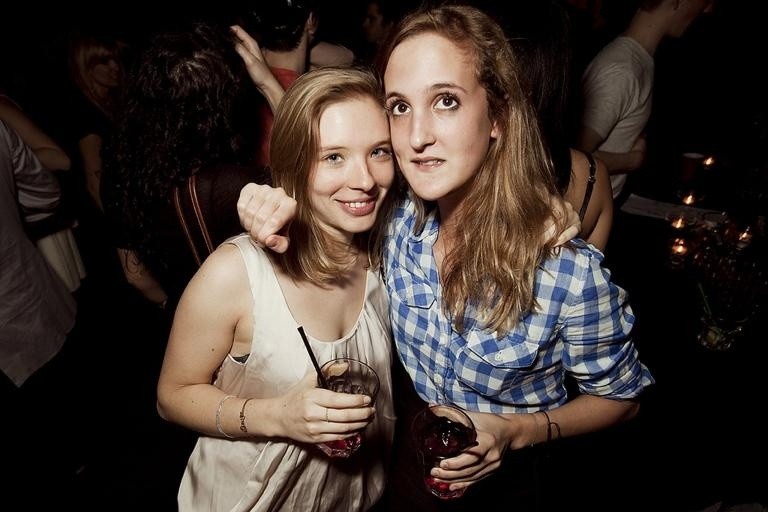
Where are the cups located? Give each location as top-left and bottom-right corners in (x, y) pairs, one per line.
(411, 405), (477, 498)
(316, 358), (380, 457)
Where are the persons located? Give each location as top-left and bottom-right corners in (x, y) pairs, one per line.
(0, 1), (409, 388)
(154, 63), (398, 511)
(234, 1), (658, 510)
(494, 1), (719, 263)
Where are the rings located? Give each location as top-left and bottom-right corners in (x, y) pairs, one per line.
(325, 406), (329, 422)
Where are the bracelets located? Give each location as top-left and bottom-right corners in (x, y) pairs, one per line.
(239, 396), (254, 434)
(535, 409), (562, 445)
(528, 414), (538, 448)
(215, 395), (238, 439)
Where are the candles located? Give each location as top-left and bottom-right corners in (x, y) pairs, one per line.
(662, 155), (753, 271)
